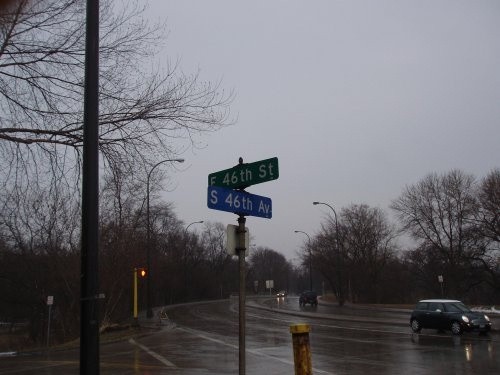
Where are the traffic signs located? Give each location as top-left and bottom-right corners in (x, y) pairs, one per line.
(208, 157), (278, 188)
(207, 186), (272, 219)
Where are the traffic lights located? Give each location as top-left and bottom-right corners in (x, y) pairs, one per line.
(137, 268), (147, 278)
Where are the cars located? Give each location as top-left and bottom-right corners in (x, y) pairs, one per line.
(277, 291), (288, 297)
(410, 299), (492, 335)
(299, 290), (318, 307)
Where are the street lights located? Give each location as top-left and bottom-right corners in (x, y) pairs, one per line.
(147, 159), (185, 313)
(185, 220), (204, 301)
(294, 231), (312, 292)
(313, 202), (339, 297)
